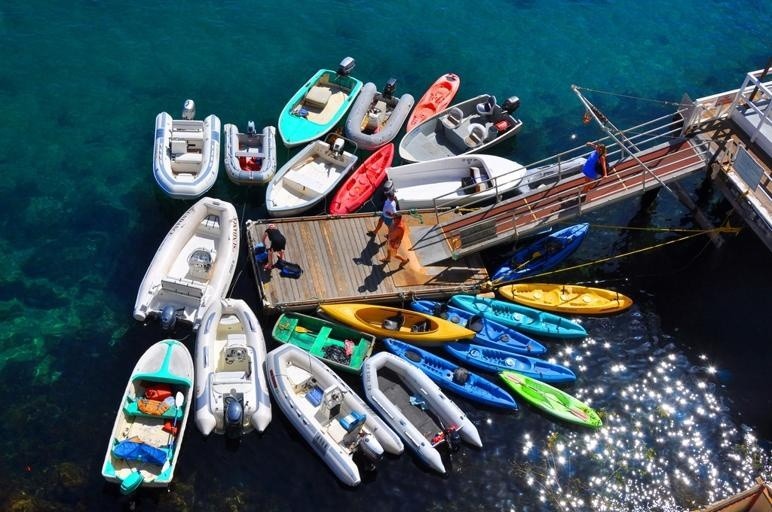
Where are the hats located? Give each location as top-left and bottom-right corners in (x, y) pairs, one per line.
(385, 190), (394, 196)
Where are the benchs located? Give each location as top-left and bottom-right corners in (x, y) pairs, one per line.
(283, 170), (329, 194)
(160, 278), (207, 299)
(173, 131), (204, 151)
(235, 151), (265, 159)
(122, 401), (183, 419)
(112, 442), (172, 463)
(310, 325), (333, 354)
(170, 153), (203, 173)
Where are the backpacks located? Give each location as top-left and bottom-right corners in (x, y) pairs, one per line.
(280, 263), (301, 279)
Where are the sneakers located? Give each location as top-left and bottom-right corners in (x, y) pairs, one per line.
(368, 231), (377, 236)
(263, 264), (274, 270)
(379, 257), (391, 262)
(399, 257), (410, 267)
(384, 233), (389, 238)
(277, 255), (286, 261)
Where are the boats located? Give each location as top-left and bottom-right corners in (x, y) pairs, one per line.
(411, 299), (547, 358)
(264, 342), (406, 486)
(223, 120), (276, 188)
(97, 338), (194, 489)
(397, 92), (525, 163)
(362, 351), (483, 474)
(271, 311), (377, 377)
(264, 133), (359, 217)
(152, 112), (221, 201)
(443, 340), (577, 387)
(498, 282), (631, 315)
(383, 153), (529, 210)
(132, 196), (240, 334)
(451, 292), (589, 340)
(277, 55), (364, 149)
(191, 297), (272, 446)
(345, 76), (416, 152)
(492, 221), (589, 285)
(405, 72), (461, 136)
(316, 302), (477, 348)
(384, 337), (519, 412)
(497, 368), (603, 433)
(328, 143), (395, 216)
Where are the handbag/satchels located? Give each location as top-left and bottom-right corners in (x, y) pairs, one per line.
(595, 154), (611, 176)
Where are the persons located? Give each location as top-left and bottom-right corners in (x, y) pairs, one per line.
(575, 141), (610, 203)
(261, 223), (287, 271)
(379, 213), (411, 268)
(366, 189), (396, 238)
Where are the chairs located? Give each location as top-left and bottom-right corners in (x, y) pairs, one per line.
(382, 315), (405, 331)
(441, 105), (464, 130)
(225, 332), (248, 347)
(463, 123), (487, 148)
(433, 303), (449, 320)
(410, 318), (431, 332)
(466, 313), (483, 333)
(340, 409), (367, 431)
